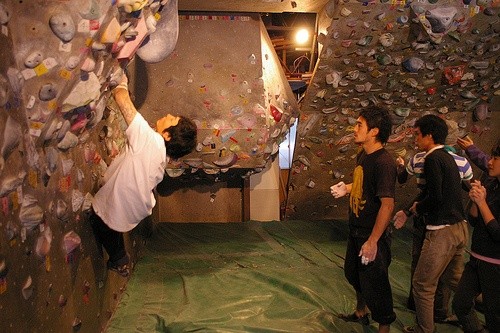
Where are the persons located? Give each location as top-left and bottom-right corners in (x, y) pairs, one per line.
(396, 119), (475, 324)
(453, 135), (500, 333)
(330, 107), (398, 333)
(88, 70), (198, 273)
(393, 114), (470, 333)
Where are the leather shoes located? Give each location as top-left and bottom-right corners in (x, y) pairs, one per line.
(339, 310), (370, 324)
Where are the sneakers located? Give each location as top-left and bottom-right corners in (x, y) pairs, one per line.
(440, 315), (459, 321)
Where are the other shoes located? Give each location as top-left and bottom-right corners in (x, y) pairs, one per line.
(404, 326), (417, 333)
(108, 260), (129, 278)
